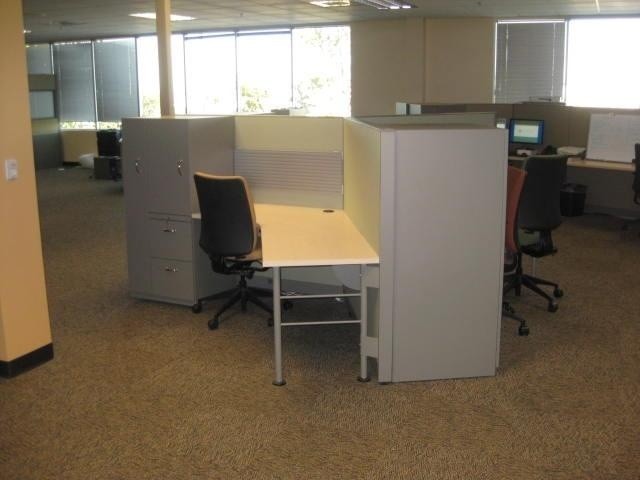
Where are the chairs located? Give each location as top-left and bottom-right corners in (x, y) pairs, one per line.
(502, 154), (568, 335)
(192, 170), (292, 329)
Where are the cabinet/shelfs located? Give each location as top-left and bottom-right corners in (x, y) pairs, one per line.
(122, 115), (236, 309)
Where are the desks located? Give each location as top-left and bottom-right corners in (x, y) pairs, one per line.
(190, 202), (379, 383)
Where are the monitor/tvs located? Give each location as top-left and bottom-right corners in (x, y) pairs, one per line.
(509, 117), (544, 150)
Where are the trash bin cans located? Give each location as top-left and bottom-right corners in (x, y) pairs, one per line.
(560, 183), (587, 217)
